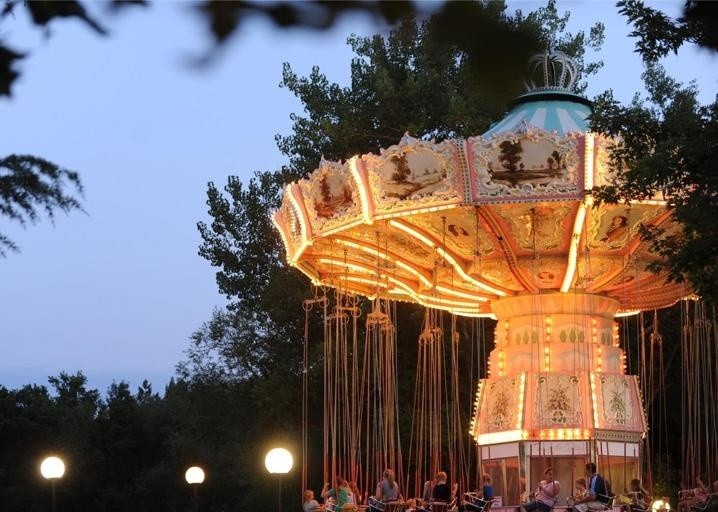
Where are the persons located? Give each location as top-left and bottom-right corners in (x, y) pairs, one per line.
(572, 462), (612, 511)
(710, 480), (718, 497)
(680, 480), (692, 500)
(302, 466), (494, 511)
(515, 466), (561, 511)
(677, 475), (711, 512)
(563, 478), (588, 511)
(620, 477), (650, 512)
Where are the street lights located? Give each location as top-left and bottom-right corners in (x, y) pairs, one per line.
(38, 456), (65, 512)
(264, 448), (293, 512)
(185, 466), (206, 511)
